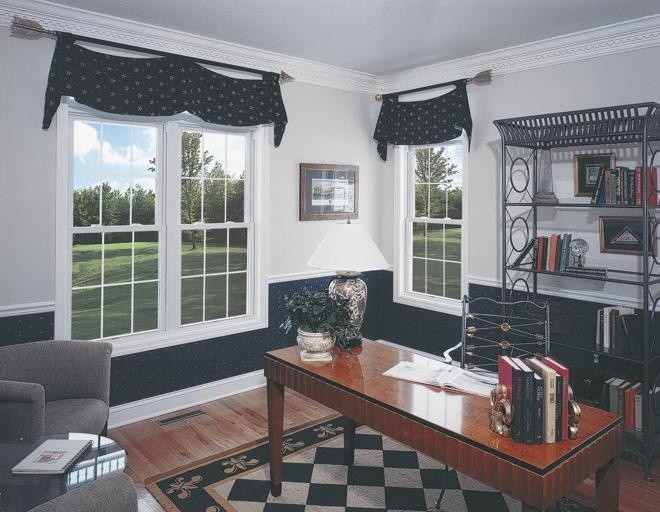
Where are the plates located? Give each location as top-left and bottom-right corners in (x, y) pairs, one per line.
(493, 102), (660, 482)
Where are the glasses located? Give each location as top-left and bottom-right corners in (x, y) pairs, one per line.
(306, 217), (391, 347)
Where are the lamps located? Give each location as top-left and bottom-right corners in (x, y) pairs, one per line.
(443, 295), (550, 378)
(0, 340), (112, 443)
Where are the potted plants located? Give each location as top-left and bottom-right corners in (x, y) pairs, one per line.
(300, 163), (359, 221)
(599, 216), (656, 255)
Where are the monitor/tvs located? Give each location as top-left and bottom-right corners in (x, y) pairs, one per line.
(514, 233), (608, 276)
(595, 304), (643, 357)
(587, 165), (657, 207)
(600, 377), (659, 436)
(383, 360), (496, 400)
(11, 439), (93, 476)
(499, 353), (569, 446)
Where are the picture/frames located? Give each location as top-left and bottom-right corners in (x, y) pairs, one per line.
(264, 337), (624, 512)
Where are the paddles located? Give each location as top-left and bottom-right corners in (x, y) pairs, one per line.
(145, 411), (596, 512)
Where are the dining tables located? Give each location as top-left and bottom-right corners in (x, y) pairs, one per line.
(278, 284), (361, 362)
